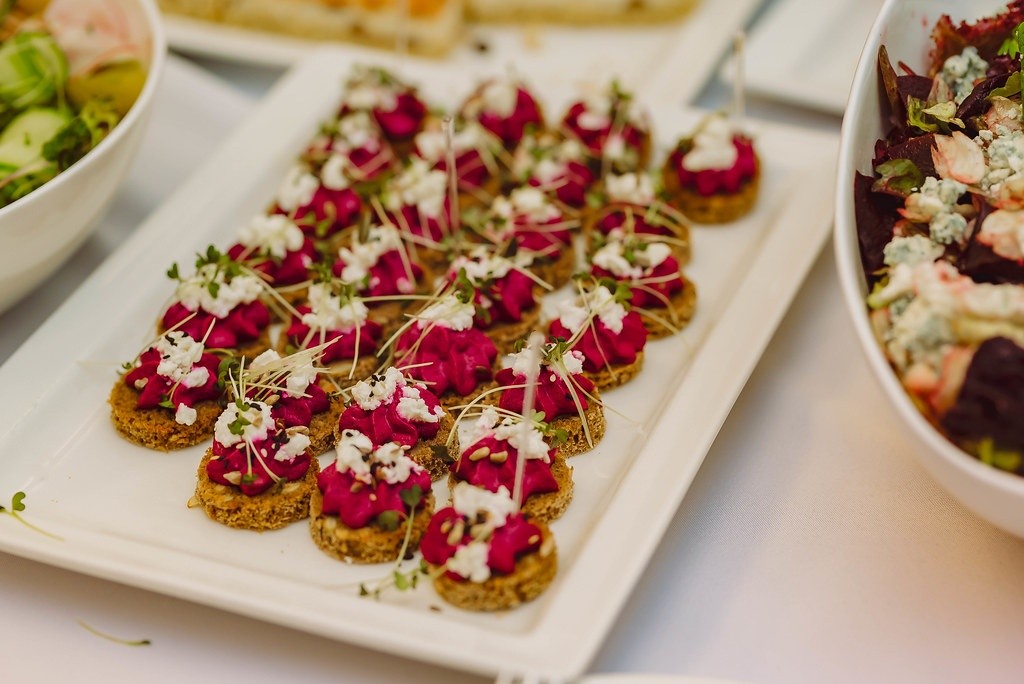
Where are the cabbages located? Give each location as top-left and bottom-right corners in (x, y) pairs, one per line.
(0, 31), (96, 211)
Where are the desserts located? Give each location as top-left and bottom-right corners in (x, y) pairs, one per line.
(110, 68), (765, 613)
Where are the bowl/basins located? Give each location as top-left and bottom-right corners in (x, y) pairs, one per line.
(0, 0), (170, 317)
(832, 0), (1024, 543)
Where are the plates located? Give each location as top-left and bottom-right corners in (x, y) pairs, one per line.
(718, 0), (888, 116)
(0, 41), (848, 684)
(157, 0), (775, 113)
